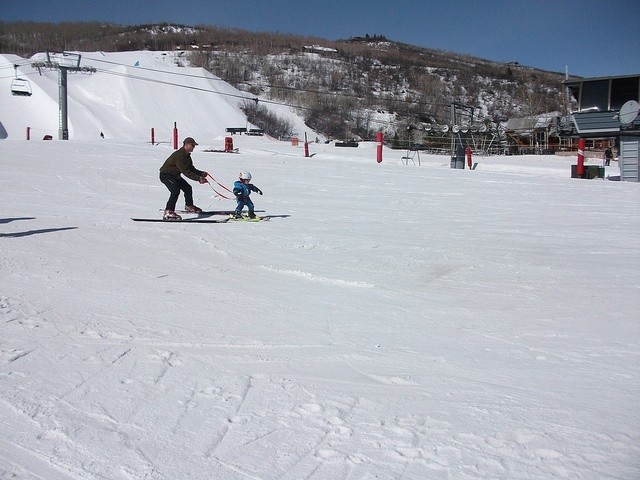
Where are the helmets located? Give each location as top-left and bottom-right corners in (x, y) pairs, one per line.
(239, 172), (251, 180)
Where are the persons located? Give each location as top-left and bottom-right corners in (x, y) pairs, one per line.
(233, 171), (263, 219)
(159, 137), (208, 221)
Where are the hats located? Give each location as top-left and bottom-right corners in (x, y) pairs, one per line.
(183, 138), (198, 145)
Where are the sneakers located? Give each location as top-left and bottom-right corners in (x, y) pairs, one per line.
(185, 205), (202, 213)
(246, 214), (256, 219)
(233, 213), (242, 219)
(163, 210), (180, 219)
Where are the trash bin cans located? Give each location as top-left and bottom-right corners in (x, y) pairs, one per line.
(450, 156), (465, 169)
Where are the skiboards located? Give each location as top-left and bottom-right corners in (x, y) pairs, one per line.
(130, 208), (246, 223)
(229, 213), (270, 222)
